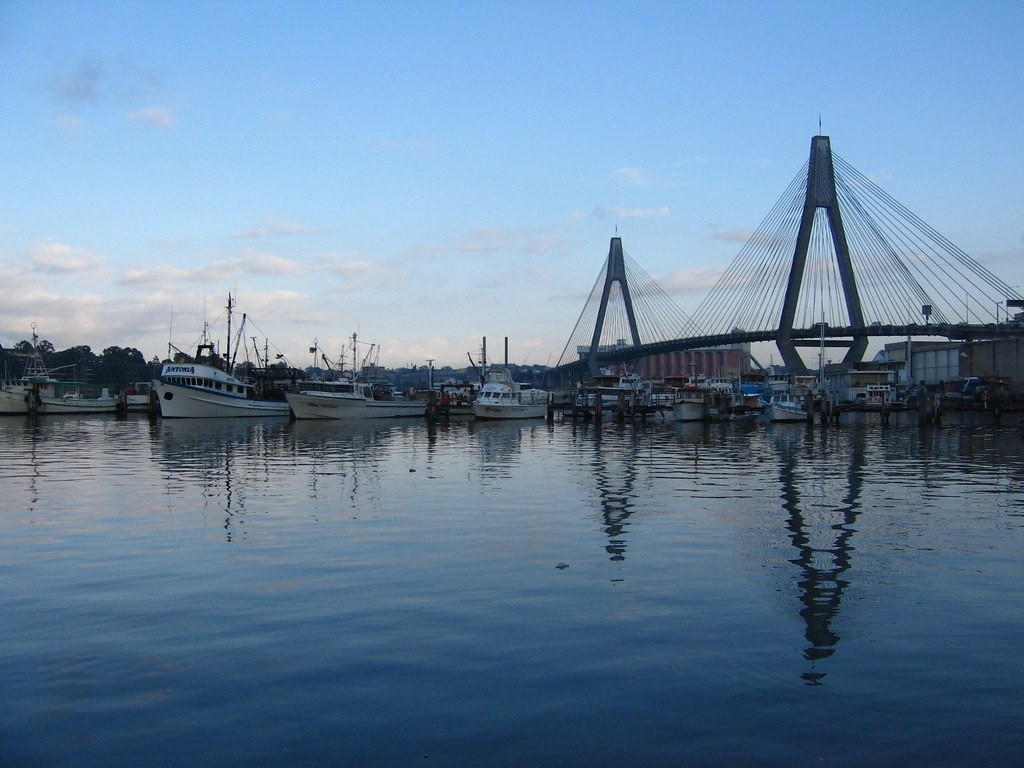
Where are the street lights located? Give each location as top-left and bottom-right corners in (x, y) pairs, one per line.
(995, 300), (1004, 326)
(425, 359), (435, 390)
(1006, 285), (1019, 324)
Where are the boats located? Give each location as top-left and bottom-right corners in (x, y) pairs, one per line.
(574, 369), (656, 407)
(719, 357), (768, 417)
(764, 383), (808, 423)
(0, 322), (126, 414)
(151, 281), (305, 419)
(668, 361), (724, 421)
(470, 367), (548, 419)
(431, 381), (478, 414)
(125, 381), (152, 411)
(281, 332), (427, 419)
